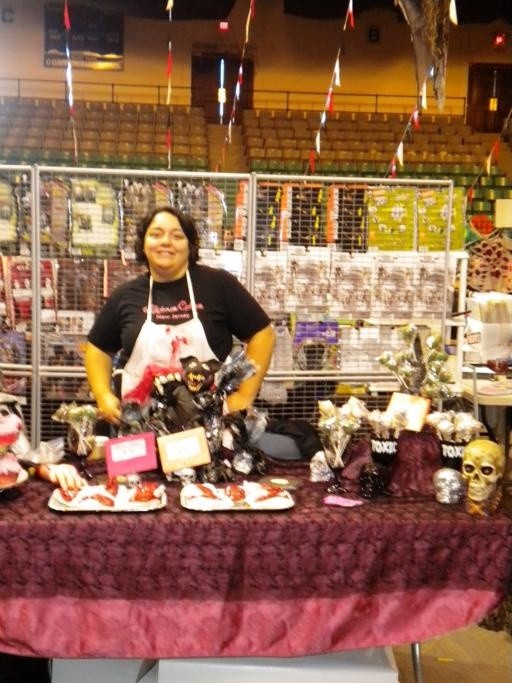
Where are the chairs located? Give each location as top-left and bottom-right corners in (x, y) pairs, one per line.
(1, 94), (209, 248)
(238, 110), (512, 248)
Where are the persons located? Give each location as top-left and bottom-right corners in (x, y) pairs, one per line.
(83, 204), (277, 428)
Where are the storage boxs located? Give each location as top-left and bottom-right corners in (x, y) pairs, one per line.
(156, 645), (397, 682)
(51, 657), (156, 682)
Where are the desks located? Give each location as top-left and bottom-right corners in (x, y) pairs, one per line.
(463, 378), (512, 446)
(0, 461), (511, 683)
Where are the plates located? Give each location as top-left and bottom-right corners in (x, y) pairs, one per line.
(179, 489), (294, 512)
(47, 484), (167, 512)
